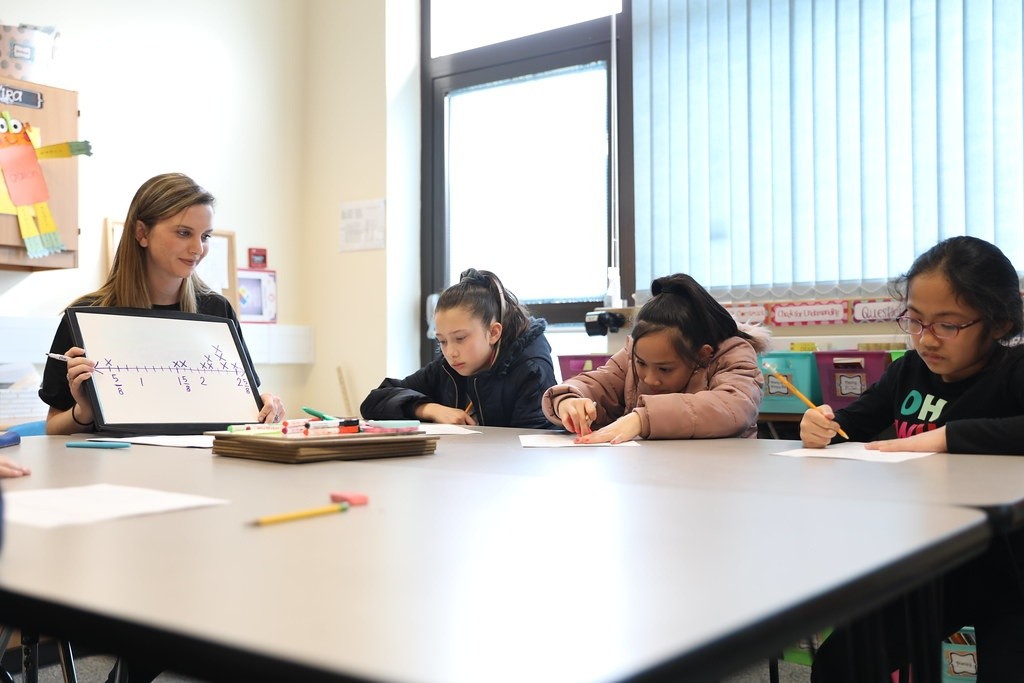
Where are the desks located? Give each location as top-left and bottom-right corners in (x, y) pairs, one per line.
(0, 422), (1024, 683)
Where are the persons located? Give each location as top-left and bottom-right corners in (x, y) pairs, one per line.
(800, 236), (1024, 683)
(39, 172), (284, 683)
(360, 268), (561, 430)
(542, 273), (771, 444)
(0, 456), (30, 683)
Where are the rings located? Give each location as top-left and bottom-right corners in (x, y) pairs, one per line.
(274, 415), (280, 421)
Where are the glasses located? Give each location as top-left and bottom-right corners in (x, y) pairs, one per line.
(895, 308), (990, 340)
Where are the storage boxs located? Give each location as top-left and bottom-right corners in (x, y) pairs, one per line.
(942, 627), (978, 683)
(782, 625), (833, 667)
(813, 348), (892, 413)
(757, 350), (824, 414)
(556, 354), (612, 382)
(890, 349), (906, 363)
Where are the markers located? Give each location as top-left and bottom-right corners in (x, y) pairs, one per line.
(304, 419), (360, 435)
(228, 419), (303, 434)
(301, 407), (337, 419)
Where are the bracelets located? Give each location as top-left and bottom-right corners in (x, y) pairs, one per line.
(72, 404), (94, 426)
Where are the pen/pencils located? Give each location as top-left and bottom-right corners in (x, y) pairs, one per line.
(585, 401), (597, 420)
(66, 441), (131, 449)
(762, 362), (849, 440)
(44, 352), (68, 362)
(244, 501), (349, 527)
(464, 402), (473, 413)
